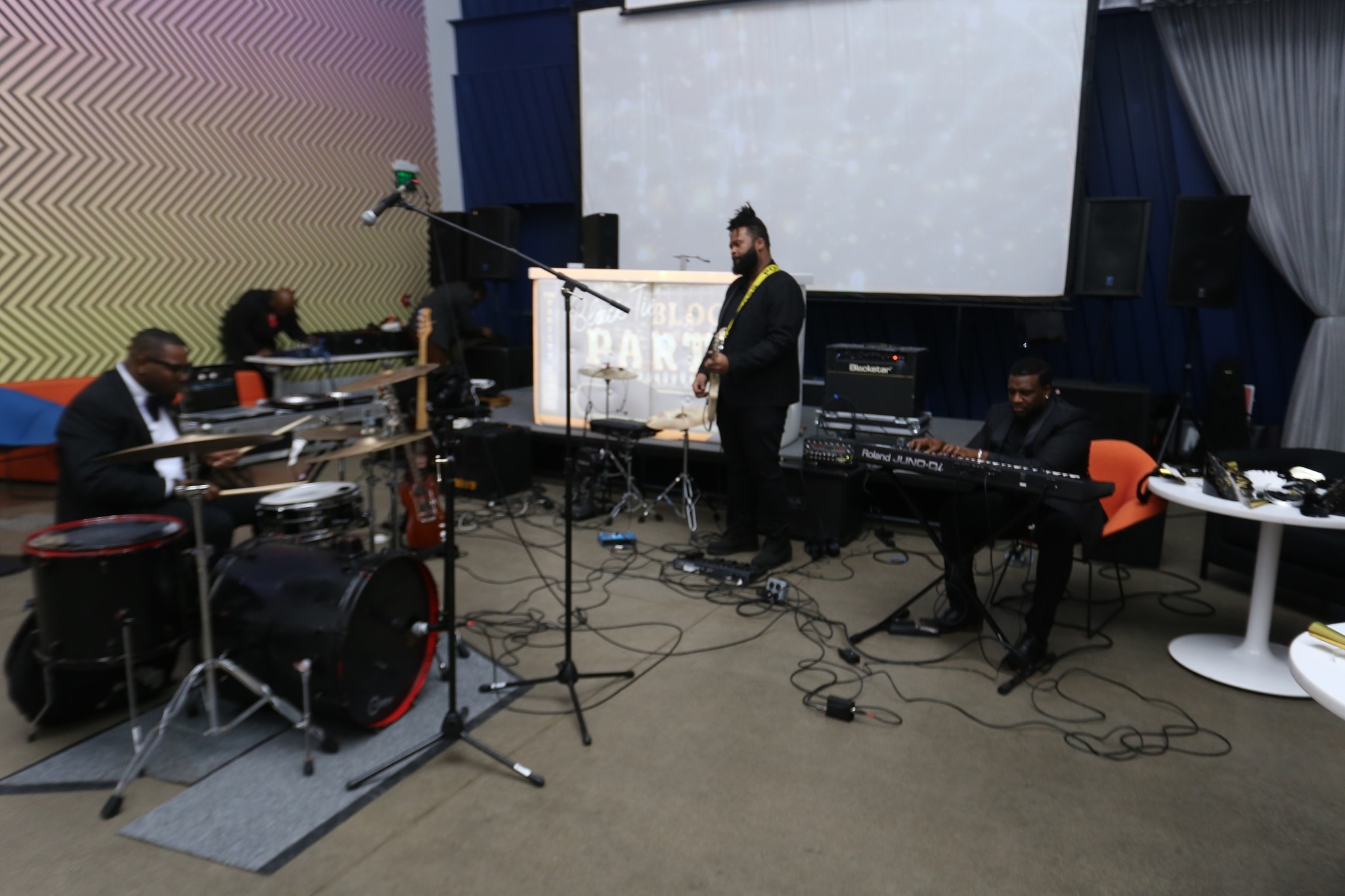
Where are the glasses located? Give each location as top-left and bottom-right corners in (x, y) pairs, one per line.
(145, 356), (193, 372)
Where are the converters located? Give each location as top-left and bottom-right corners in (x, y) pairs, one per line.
(825, 695), (854, 723)
(599, 531), (636, 548)
(684, 550), (704, 560)
(839, 648), (860, 666)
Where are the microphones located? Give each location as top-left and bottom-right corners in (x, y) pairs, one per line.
(359, 185), (407, 227)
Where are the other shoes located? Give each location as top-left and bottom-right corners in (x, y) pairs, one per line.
(751, 536), (794, 569)
(705, 528), (760, 555)
(929, 601), (987, 633)
(1005, 628), (1051, 672)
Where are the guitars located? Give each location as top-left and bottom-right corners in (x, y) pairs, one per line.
(707, 324), (728, 421)
(380, 383), (447, 550)
(398, 307), (438, 535)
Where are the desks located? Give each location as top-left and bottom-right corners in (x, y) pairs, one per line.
(1145, 467), (1345, 702)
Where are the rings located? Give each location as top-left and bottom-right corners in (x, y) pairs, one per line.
(956, 445), (960, 451)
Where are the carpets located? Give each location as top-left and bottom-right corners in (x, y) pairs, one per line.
(0, 637), (538, 877)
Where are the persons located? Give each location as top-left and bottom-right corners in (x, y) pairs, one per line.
(904, 356), (1091, 672)
(692, 203), (809, 569)
(222, 287), (326, 363)
(409, 279), (494, 370)
(52, 327), (262, 570)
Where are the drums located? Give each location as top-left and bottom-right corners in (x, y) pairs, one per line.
(254, 480), (370, 557)
(22, 512), (198, 665)
(200, 531), (440, 736)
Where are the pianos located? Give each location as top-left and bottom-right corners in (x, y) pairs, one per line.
(847, 441), (1116, 695)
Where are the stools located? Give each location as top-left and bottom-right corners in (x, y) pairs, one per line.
(590, 417), (663, 524)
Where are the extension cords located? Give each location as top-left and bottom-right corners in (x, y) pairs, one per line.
(766, 576), (788, 603)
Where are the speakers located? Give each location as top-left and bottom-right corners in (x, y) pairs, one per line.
(465, 205), (519, 280)
(428, 212), (466, 284)
(1166, 194), (1251, 311)
(1075, 195), (1152, 299)
(583, 212), (619, 269)
(450, 421), (533, 502)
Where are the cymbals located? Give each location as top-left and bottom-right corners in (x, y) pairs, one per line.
(331, 361), (440, 393)
(578, 365), (638, 381)
(294, 425), (383, 439)
(94, 431), (283, 467)
(303, 427), (433, 463)
(645, 406), (710, 430)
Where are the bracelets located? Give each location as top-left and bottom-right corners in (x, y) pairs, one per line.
(976, 447), (984, 460)
